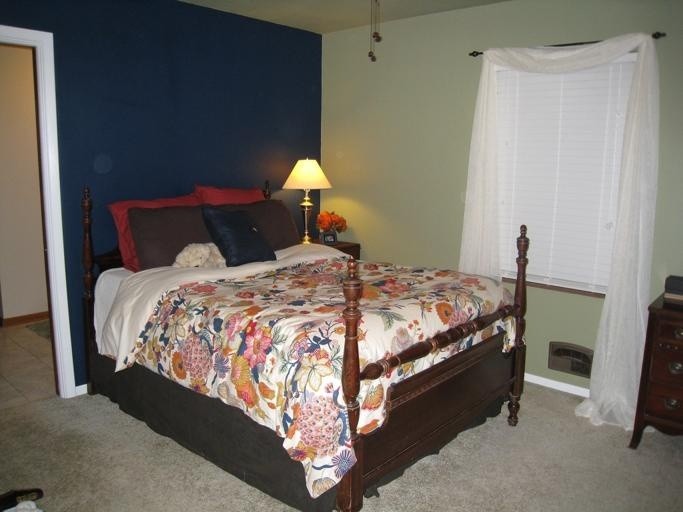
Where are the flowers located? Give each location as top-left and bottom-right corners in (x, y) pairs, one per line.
(315, 209), (347, 232)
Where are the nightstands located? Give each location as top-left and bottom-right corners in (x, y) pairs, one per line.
(313, 235), (360, 262)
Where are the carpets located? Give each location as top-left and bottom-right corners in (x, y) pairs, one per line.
(1, 377), (683, 511)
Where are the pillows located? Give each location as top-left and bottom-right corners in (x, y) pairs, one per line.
(127, 198), (300, 269)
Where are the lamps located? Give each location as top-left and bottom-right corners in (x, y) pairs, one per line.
(282, 155), (327, 248)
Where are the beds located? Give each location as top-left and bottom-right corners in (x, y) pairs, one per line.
(79, 178), (532, 510)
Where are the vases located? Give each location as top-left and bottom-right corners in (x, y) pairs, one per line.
(320, 230), (338, 242)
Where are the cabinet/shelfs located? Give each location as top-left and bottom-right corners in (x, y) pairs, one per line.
(629, 294), (683, 456)
